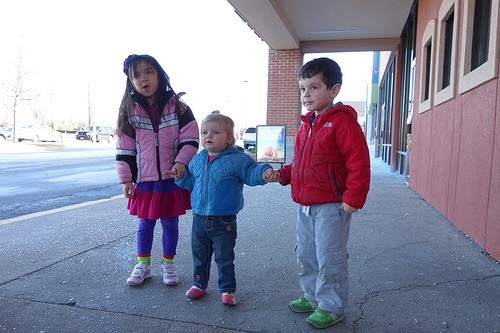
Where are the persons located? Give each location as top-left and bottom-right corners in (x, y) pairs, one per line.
(115, 55), (199, 286)
(263, 57), (371, 329)
(162, 110), (280, 306)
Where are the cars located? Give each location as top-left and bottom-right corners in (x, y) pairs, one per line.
(0, 120), (65, 143)
(75, 125), (116, 143)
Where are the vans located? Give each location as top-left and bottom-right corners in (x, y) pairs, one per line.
(243, 126), (257, 149)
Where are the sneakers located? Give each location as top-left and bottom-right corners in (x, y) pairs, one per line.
(288, 295), (318, 313)
(306, 307), (345, 329)
(160, 261), (180, 285)
(126, 263), (153, 285)
(221, 292), (237, 305)
(186, 285), (209, 299)
